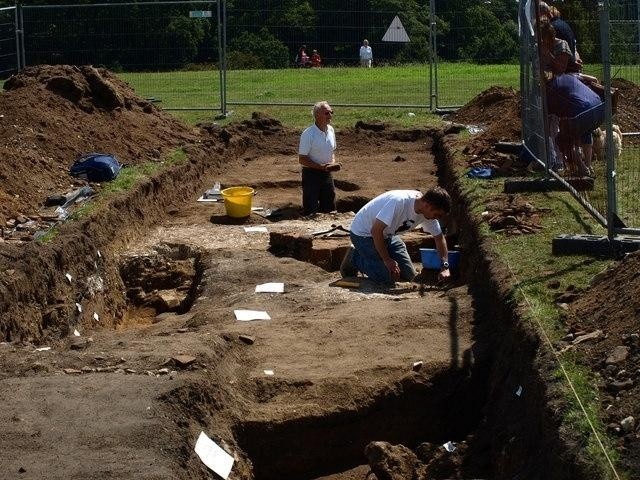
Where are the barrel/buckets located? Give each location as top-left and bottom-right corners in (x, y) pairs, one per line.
(220, 187), (254, 218)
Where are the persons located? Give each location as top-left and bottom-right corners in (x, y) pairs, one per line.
(340, 186), (455, 285)
(543, 72), (602, 175)
(537, 1), (585, 71)
(298, 100), (344, 217)
(535, 16), (573, 173)
(296, 44), (310, 66)
(549, 7), (560, 18)
(310, 49), (321, 66)
(357, 38), (373, 67)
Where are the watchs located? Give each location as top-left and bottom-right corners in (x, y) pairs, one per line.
(438, 261), (450, 270)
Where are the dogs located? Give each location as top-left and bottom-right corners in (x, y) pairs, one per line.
(592, 124), (624, 160)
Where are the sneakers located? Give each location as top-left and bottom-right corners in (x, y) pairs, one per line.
(554, 163), (596, 177)
(338, 244), (360, 280)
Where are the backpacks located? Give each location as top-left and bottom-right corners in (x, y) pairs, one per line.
(70, 151), (121, 181)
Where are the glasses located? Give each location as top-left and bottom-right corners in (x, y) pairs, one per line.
(319, 109), (333, 115)
